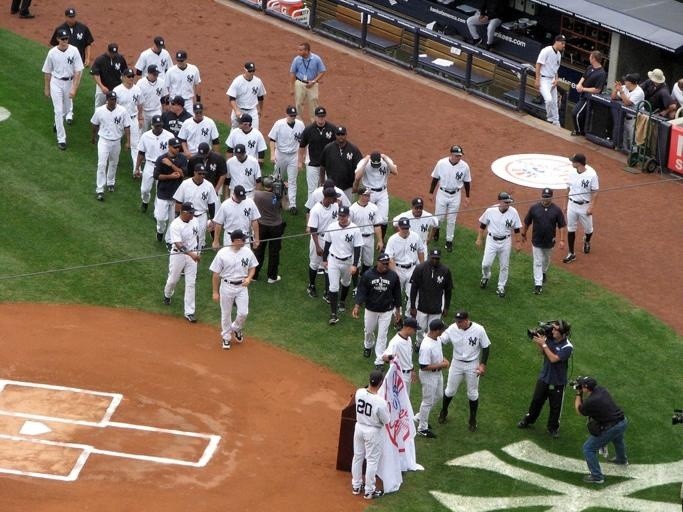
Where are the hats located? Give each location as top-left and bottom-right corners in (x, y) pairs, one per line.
(313, 106), (326, 114)
(555, 35), (567, 41)
(450, 145), (464, 157)
(65, 8), (77, 16)
(621, 73), (637, 84)
(334, 126), (348, 139)
(105, 36), (209, 212)
(58, 29), (68, 40)
(403, 311), (470, 331)
(497, 191), (514, 203)
(369, 371), (384, 385)
(647, 69), (665, 83)
(569, 153), (585, 165)
(229, 106), (299, 240)
(244, 62), (255, 73)
(542, 188), (553, 196)
(311, 183), (442, 272)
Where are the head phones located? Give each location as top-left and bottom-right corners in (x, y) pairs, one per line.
(559, 320), (566, 336)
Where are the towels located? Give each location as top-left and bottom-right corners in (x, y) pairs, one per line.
(432, 57), (454, 67)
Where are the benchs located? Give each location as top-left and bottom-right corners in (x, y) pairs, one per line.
(369, 0), (556, 64)
(503, 74), (570, 122)
(415, 39), (495, 94)
(320, 4), (404, 59)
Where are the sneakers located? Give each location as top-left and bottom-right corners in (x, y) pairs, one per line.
(479, 278), (488, 288)
(532, 272), (546, 294)
(563, 252), (576, 263)
(375, 365), (384, 373)
(184, 314), (197, 322)
(164, 294), (171, 305)
(307, 284), (345, 324)
(582, 446), (627, 484)
(233, 331), (244, 343)
(420, 413), (558, 437)
(222, 339), (231, 349)
(495, 288), (505, 296)
(582, 236), (591, 254)
(353, 481), (383, 497)
(363, 347), (371, 357)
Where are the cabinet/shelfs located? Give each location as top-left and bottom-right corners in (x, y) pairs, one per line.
(559, 15), (611, 71)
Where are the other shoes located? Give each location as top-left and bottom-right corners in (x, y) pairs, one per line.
(59, 143), (67, 150)
(97, 193), (104, 201)
(445, 240), (453, 253)
(157, 232), (164, 242)
(66, 119), (72, 125)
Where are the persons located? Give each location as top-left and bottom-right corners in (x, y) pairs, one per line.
(525, 185), (570, 291)
(91, 33), (219, 241)
(640, 68), (676, 118)
(10, 0), (38, 20)
(477, 189), (522, 297)
(669, 76), (683, 116)
(533, 32), (570, 125)
(374, 315), (420, 404)
(435, 312), (491, 432)
(572, 376), (632, 484)
(466, 2), (514, 49)
(570, 45), (607, 134)
(351, 372), (392, 501)
(610, 69), (646, 143)
(412, 317), (451, 439)
(162, 165), (262, 348)
(52, 8), (94, 71)
(304, 143), (473, 372)
(562, 150), (600, 268)
(223, 43), (364, 284)
(517, 319), (573, 438)
(42, 30), (85, 153)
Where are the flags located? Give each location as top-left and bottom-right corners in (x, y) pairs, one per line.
(364, 360), (429, 494)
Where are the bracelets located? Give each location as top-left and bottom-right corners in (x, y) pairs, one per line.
(521, 232), (526, 237)
(541, 342), (548, 348)
(575, 393), (581, 396)
(516, 240), (520, 243)
(619, 91), (625, 94)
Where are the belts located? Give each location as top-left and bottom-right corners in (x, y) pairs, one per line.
(488, 233), (510, 240)
(440, 187), (460, 195)
(224, 279), (242, 286)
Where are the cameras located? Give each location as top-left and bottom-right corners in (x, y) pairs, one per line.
(569, 375), (584, 392)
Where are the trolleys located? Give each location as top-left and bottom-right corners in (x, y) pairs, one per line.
(626, 99), (658, 174)
